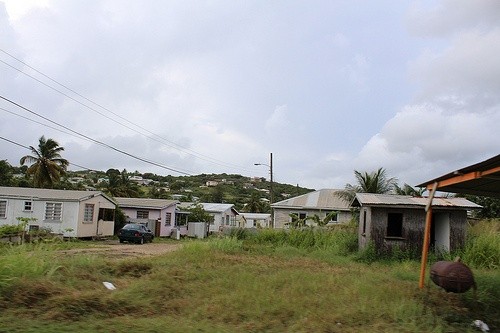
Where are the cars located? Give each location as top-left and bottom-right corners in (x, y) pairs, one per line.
(117, 224), (154, 245)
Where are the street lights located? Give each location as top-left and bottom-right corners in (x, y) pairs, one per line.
(254, 152), (274, 228)
